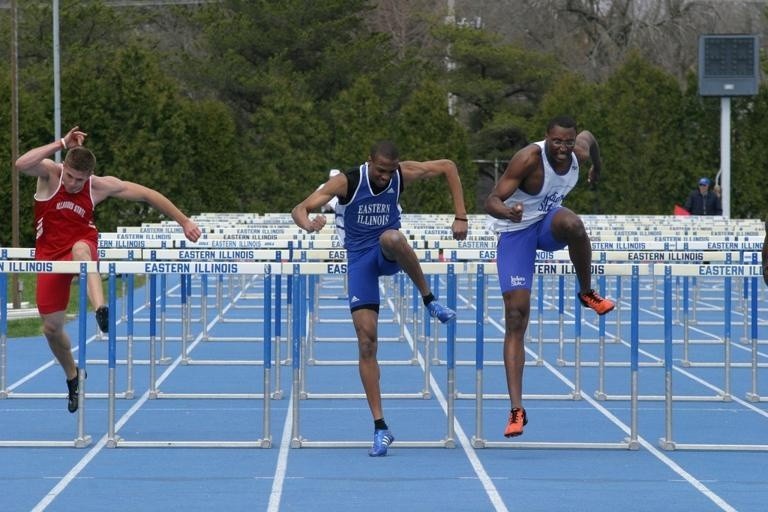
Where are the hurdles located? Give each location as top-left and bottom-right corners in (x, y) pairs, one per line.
(99, 261), (282, 448)
(743, 250), (768, 404)
(443, 249), (584, 401)
(142, 248), (291, 402)
(653, 263), (768, 452)
(592, 249), (740, 401)
(0, 259), (98, 451)
(467, 262), (650, 450)
(93, 212), (768, 367)
(0, 248), (141, 400)
(282, 262), (465, 449)
(291, 247), (441, 400)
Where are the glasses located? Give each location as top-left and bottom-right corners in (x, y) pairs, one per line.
(699, 184), (707, 186)
(547, 132), (577, 148)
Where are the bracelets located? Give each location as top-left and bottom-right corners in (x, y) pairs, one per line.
(59, 136), (69, 152)
(454, 216), (469, 222)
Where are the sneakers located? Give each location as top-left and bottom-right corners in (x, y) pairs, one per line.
(578, 289), (615, 316)
(66, 366), (87, 412)
(368, 429), (395, 456)
(427, 300), (457, 324)
(95, 305), (109, 334)
(504, 406), (528, 436)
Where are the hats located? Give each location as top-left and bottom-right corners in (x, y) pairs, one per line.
(698, 178), (711, 186)
(329, 169), (341, 177)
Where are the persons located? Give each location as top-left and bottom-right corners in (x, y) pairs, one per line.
(481, 115), (620, 439)
(289, 141), (469, 457)
(316, 167), (344, 213)
(682, 175), (723, 215)
(12, 123), (202, 412)
